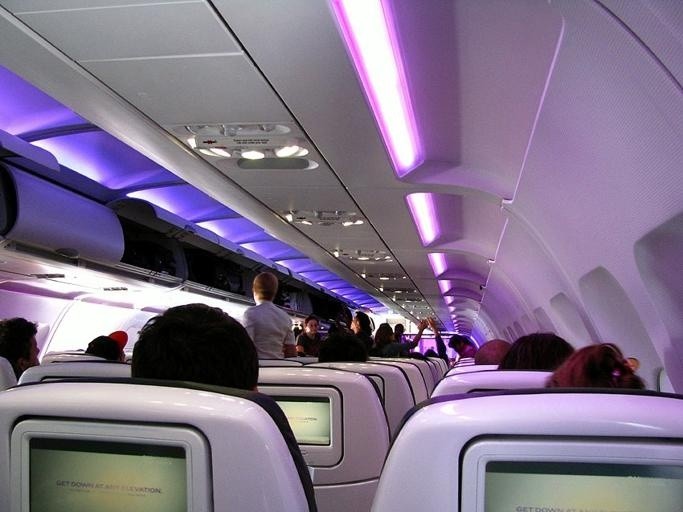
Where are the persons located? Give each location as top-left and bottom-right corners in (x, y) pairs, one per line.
(0, 316), (40, 383)
(545, 342), (646, 389)
(496, 333), (575, 372)
(242, 272), (297, 359)
(130, 302), (259, 392)
(292, 310), (510, 379)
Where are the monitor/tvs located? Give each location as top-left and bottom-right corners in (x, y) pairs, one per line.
(24, 439), (191, 512)
(481, 458), (683, 512)
(273, 397), (331, 447)
